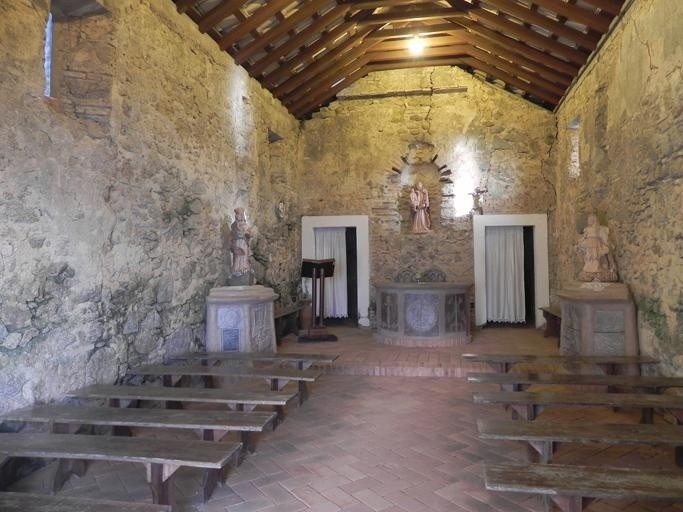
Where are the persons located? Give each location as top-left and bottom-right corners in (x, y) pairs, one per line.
(584, 214), (609, 273)
(410, 182), (430, 234)
(231, 207), (251, 274)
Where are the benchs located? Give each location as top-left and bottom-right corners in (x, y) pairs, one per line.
(460, 353), (683, 512)
(3, 352), (341, 511)
